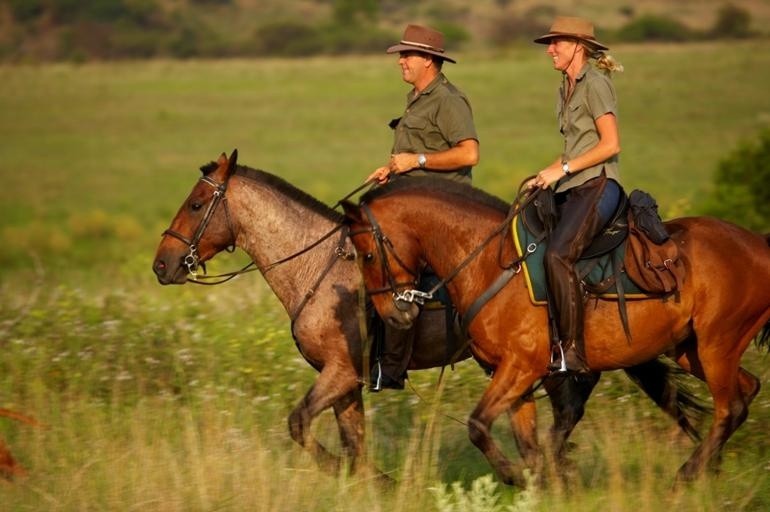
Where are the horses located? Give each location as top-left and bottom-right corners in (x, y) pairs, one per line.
(151, 147), (714, 484)
(337, 174), (770, 494)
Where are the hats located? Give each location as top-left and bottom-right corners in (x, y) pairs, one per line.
(386, 24), (456, 64)
(533, 15), (610, 52)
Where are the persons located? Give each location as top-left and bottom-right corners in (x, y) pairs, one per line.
(522, 13), (625, 376)
(353, 18), (480, 390)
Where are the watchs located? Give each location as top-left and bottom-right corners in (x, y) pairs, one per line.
(562, 158), (570, 179)
(418, 150), (427, 168)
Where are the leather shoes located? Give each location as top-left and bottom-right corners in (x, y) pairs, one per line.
(549, 352), (592, 377)
(371, 374), (404, 390)
